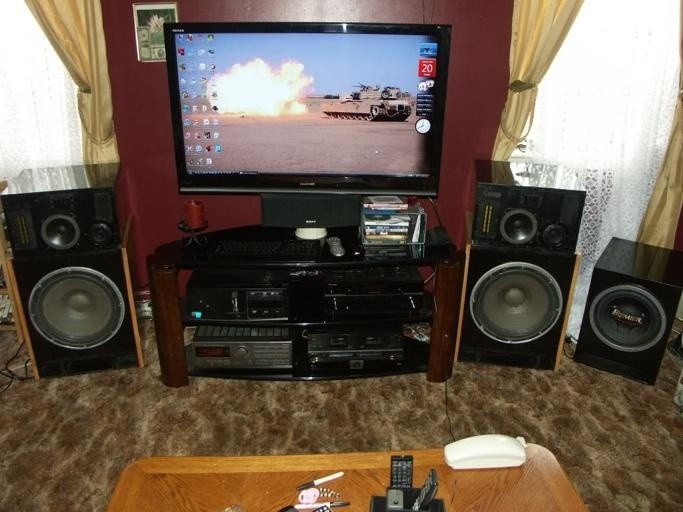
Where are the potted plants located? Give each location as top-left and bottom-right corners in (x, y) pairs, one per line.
(130, 1), (181, 65)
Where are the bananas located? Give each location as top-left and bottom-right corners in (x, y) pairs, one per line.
(213, 240), (321, 261)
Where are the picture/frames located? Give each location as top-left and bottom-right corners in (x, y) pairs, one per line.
(146, 225), (466, 387)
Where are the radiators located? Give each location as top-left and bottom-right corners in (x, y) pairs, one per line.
(411, 469), (438, 512)
(326, 237), (345, 257)
(390, 455), (413, 489)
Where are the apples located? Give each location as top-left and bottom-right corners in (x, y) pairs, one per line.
(185, 199), (205, 227)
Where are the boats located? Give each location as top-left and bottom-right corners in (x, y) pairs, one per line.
(0, 163), (131, 257)
(454, 212), (581, 371)
(466, 158), (586, 252)
(574, 237), (683, 386)
(262, 192), (361, 228)
(6, 214), (145, 380)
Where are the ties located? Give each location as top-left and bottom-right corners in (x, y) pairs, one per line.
(164, 22), (452, 197)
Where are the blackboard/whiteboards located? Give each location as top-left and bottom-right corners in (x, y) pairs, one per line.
(302, 83), (412, 121)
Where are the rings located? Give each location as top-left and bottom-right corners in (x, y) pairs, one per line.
(296, 471), (345, 491)
(293, 501), (351, 509)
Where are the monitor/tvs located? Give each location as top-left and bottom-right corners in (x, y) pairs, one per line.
(359, 196), (411, 257)
